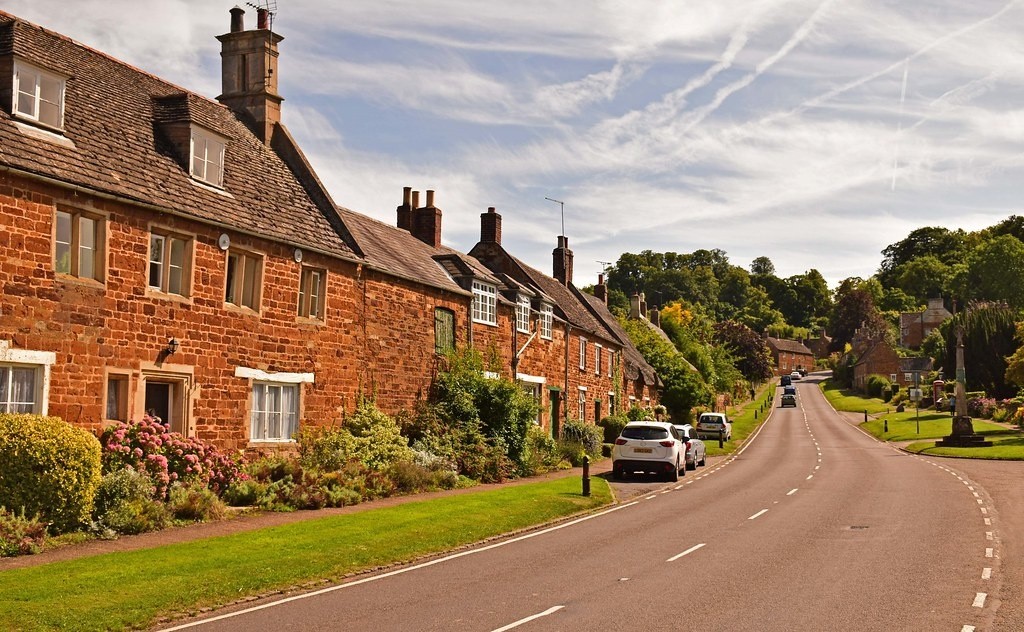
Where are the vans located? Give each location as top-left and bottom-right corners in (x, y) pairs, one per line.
(780, 375), (792, 386)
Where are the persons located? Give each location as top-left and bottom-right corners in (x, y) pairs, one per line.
(950, 395), (956, 416)
(801, 368), (804, 377)
(749, 388), (755, 401)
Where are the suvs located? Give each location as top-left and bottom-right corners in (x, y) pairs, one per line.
(674, 423), (707, 471)
(612, 420), (690, 483)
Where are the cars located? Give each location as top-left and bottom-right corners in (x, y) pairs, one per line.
(790, 371), (802, 380)
(695, 413), (734, 442)
(783, 384), (797, 395)
(797, 369), (809, 377)
(780, 394), (797, 408)
